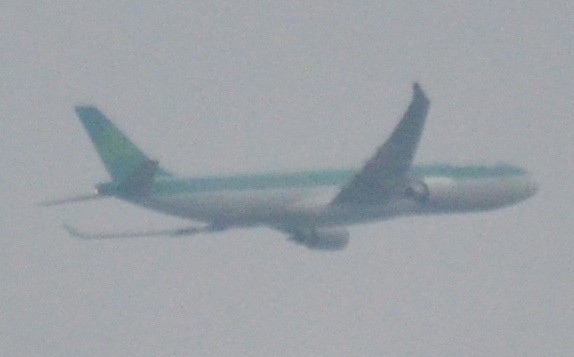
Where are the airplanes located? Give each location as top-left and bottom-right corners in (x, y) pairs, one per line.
(38, 82), (539, 250)
